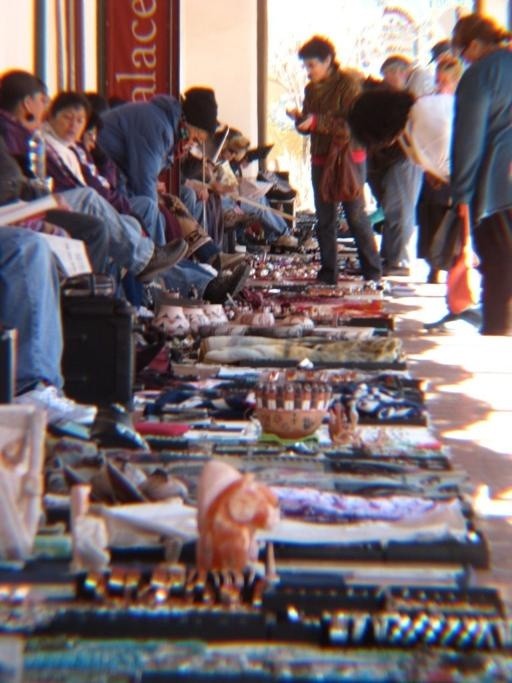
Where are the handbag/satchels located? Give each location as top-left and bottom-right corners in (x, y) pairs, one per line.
(318, 137), (358, 201)
(425, 208), (463, 269)
(447, 261), (475, 314)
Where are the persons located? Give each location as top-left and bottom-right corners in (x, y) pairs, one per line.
(0, 66), (300, 429)
(285, 12), (512, 336)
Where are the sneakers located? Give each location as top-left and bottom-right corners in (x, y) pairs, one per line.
(15, 382), (97, 425)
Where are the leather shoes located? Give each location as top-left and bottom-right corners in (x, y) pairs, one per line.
(204, 259), (250, 303)
(137, 238), (189, 283)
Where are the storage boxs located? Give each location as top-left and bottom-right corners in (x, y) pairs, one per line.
(60, 294), (131, 404)
